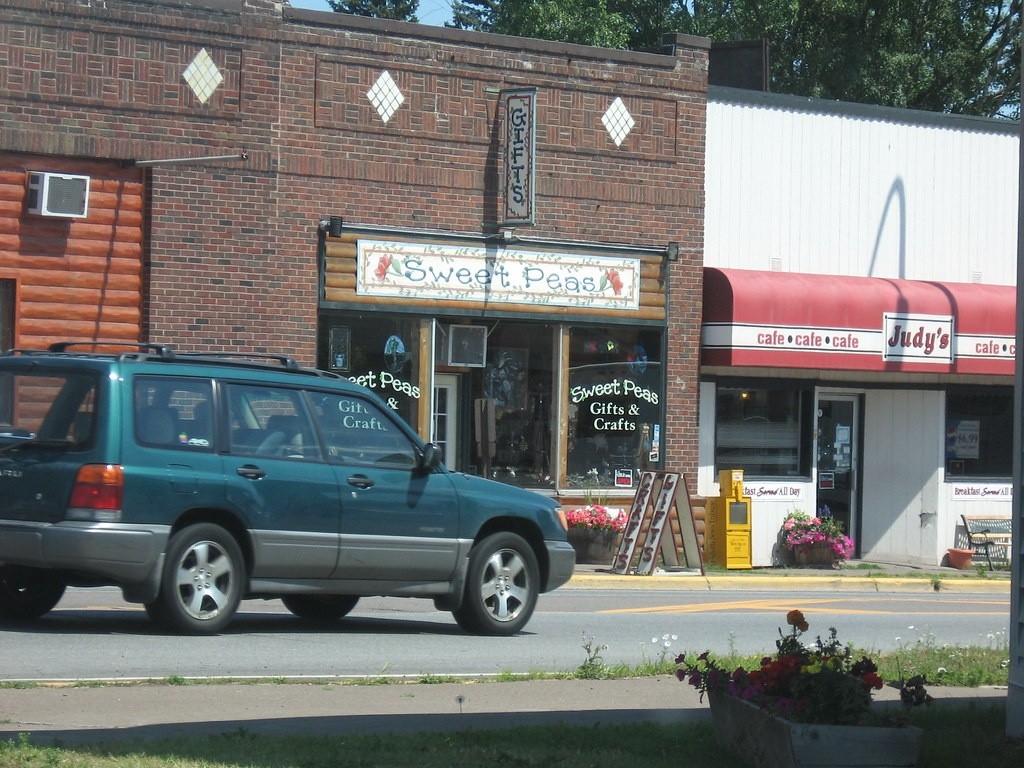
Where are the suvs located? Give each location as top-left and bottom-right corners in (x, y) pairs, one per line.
(0, 342), (576, 636)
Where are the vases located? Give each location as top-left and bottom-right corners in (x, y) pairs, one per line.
(702, 679), (924, 768)
(946, 548), (977, 570)
(563, 526), (621, 566)
(792, 542), (836, 566)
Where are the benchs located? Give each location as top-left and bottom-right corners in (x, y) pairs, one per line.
(960, 513), (1012, 571)
(71, 405), (180, 446)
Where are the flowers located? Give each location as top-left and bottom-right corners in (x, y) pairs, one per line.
(565, 505), (630, 533)
(674, 610), (937, 725)
(775, 502), (855, 561)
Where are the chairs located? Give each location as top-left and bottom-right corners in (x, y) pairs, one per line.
(191, 399), (214, 445)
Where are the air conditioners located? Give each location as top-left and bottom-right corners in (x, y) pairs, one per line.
(26, 171), (92, 219)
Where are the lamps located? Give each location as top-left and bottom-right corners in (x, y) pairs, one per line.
(664, 241), (679, 262)
(323, 214), (344, 238)
